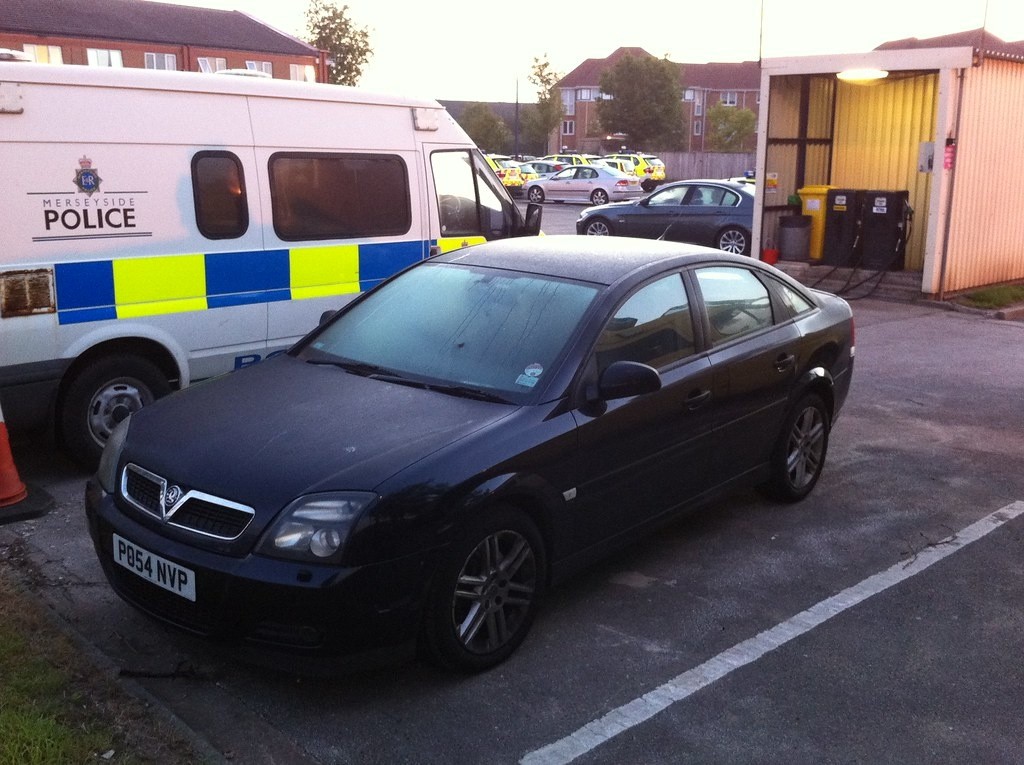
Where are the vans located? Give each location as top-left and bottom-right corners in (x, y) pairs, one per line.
(0, 52), (544, 475)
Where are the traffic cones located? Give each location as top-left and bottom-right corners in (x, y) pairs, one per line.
(0, 403), (57, 524)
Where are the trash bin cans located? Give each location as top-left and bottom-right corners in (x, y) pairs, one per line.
(779, 214), (813, 261)
(796, 184), (840, 259)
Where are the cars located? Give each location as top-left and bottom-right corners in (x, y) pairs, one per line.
(482, 145), (668, 206)
(658, 176), (756, 206)
(83, 234), (858, 678)
(575, 179), (756, 257)
